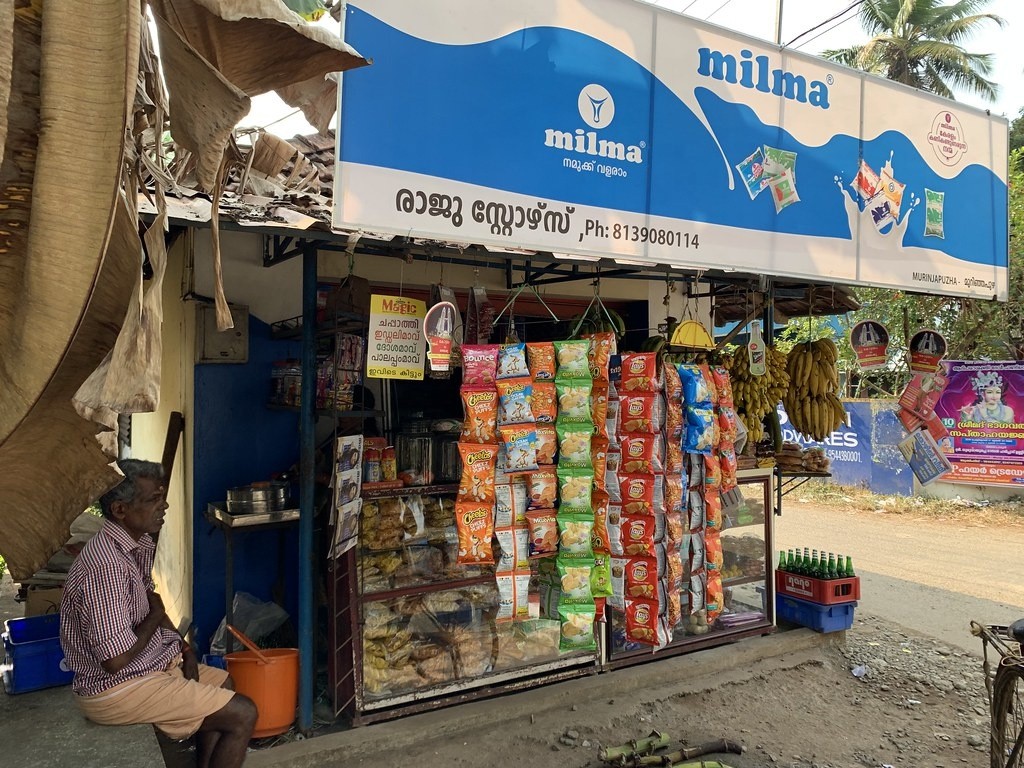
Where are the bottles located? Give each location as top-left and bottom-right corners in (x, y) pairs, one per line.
(777, 547), (856, 596)
(269, 360), (302, 406)
(748, 322), (766, 376)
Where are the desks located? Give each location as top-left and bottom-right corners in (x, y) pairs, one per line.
(202, 511), (330, 710)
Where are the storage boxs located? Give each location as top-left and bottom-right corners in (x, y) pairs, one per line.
(26, 586), (62, 617)
(2, 613), (75, 695)
(774, 570), (861, 633)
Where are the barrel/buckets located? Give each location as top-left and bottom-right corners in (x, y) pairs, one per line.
(223, 624), (299, 738)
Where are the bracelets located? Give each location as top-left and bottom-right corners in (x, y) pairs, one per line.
(180, 640), (191, 653)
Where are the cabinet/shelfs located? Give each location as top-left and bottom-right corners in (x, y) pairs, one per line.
(272, 306), (778, 727)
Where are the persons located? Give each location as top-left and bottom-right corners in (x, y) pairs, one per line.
(337, 512), (359, 552)
(959, 371), (1015, 426)
(269, 384), (381, 522)
(338, 445), (358, 472)
(60, 458), (258, 768)
(340, 479), (357, 506)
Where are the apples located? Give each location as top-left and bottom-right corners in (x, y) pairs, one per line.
(720, 564), (743, 578)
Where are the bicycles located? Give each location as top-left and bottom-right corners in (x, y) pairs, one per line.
(970, 618), (1024, 768)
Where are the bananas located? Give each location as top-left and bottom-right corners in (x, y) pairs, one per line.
(567, 306), (626, 346)
(644, 335), (849, 441)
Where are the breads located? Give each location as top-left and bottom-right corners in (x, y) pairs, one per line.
(359, 498), (559, 692)
(775, 441), (830, 473)
(686, 609), (710, 634)
